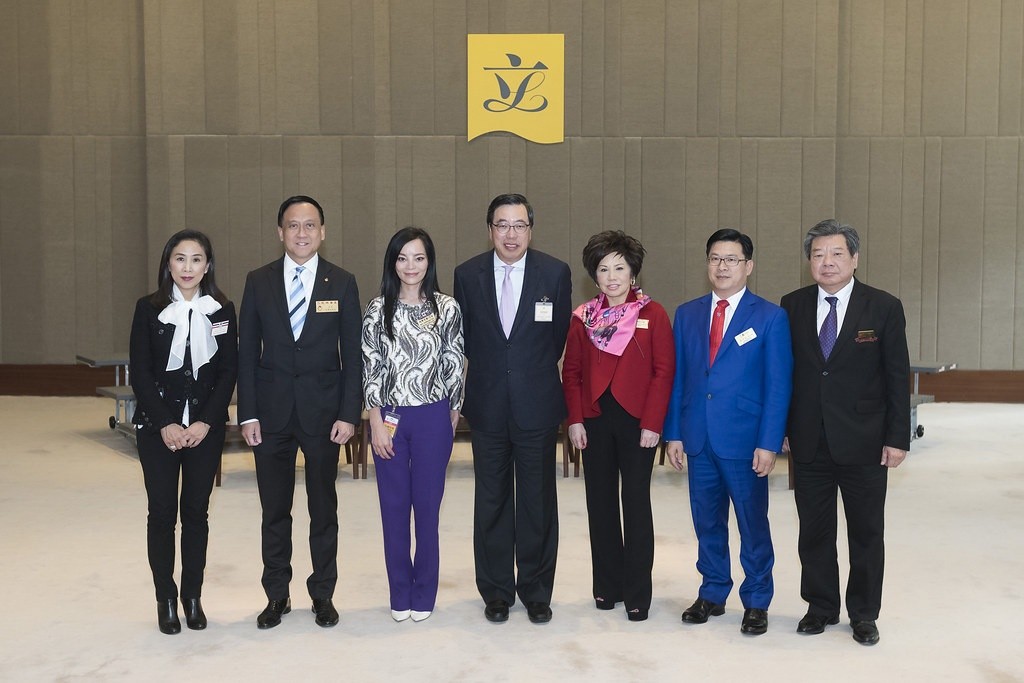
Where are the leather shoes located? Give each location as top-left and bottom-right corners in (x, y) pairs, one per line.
(849, 618), (880, 646)
(682, 601), (726, 624)
(485, 602), (509, 622)
(526, 601), (553, 624)
(740, 609), (769, 635)
(257, 598), (291, 629)
(797, 613), (840, 635)
(311, 600), (340, 627)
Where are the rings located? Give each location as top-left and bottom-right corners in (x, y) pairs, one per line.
(170, 445), (176, 449)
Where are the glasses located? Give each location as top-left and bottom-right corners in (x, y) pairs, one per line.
(706, 256), (748, 267)
(492, 221), (531, 234)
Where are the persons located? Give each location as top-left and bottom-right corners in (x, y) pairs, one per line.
(361, 225), (465, 622)
(454, 193), (572, 626)
(780, 219), (911, 646)
(562, 230), (675, 621)
(663, 229), (790, 634)
(236, 194), (362, 630)
(128, 228), (238, 636)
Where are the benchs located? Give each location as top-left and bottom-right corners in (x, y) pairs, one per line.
(95, 386), (137, 400)
(909, 394), (935, 408)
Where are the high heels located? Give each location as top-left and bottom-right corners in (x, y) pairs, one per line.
(391, 609), (431, 623)
(156, 598), (208, 634)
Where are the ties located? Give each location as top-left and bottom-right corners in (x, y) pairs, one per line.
(498, 265), (517, 339)
(709, 299), (729, 367)
(289, 267), (307, 342)
(818, 297), (837, 360)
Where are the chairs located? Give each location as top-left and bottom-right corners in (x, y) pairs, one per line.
(216, 334), (796, 491)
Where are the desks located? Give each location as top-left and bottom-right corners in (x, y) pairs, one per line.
(76, 354), (132, 429)
(909, 358), (959, 442)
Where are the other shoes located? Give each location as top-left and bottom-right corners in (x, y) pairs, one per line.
(596, 598), (648, 622)
